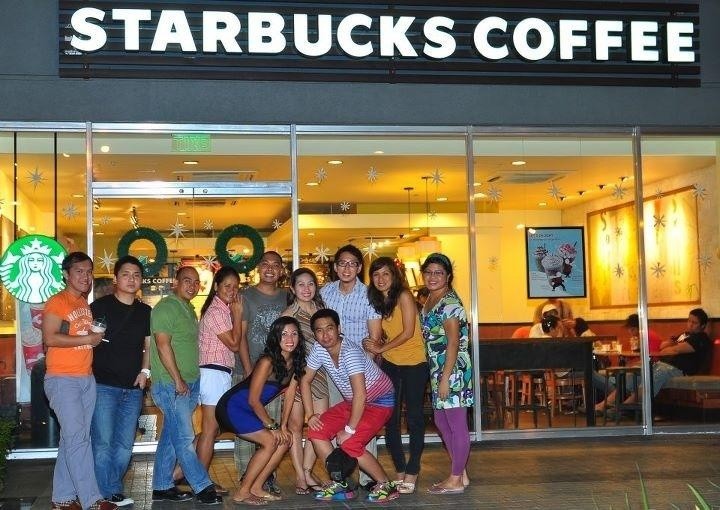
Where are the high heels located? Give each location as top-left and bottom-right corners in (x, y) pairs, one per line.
(398, 482), (418, 494)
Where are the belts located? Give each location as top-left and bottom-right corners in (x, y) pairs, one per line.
(201, 362), (232, 371)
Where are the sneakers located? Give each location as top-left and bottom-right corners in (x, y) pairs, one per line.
(107, 493), (134, 507)
(51, 496), (115, 509)
(363, 482), (400, 502)
(311, 482), (358, 500)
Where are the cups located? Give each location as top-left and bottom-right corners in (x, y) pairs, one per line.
(89, 319), (106, 348)
(533, 252), (576, 287)
(594, 341), (622, 353)
(20, 304), (45, 378)
(629, 337), (639, 350)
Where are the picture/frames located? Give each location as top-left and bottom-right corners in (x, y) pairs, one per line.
(524, 226), (587, 299)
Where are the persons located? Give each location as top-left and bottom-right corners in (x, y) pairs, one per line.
(41, 245), (476, 508)
(527, 303), (605, 416)
(595, 306), (714, 420)
(575, 313), (666, 418)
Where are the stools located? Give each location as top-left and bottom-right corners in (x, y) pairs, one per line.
(517, 372), (546, 414)
(470, 365), (501, 429)
(505, 369), (552, 429)
(604, 366), (640, 426)
(545, 370), (586, 417)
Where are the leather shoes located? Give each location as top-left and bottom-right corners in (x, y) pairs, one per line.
(150, 488), (193, 504)
(195, 485), (224, 506)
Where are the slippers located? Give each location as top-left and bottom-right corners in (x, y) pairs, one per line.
(233, 490), (283, 505)
(294, 481), (322, 494)
(425, 484), (467, 496)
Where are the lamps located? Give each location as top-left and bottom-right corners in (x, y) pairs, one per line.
(397, 187), (420, 268)
(91, 180), (292, 200)
(415, 176), (442, 259)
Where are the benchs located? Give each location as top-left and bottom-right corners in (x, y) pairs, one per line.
(661, 337), (720, 419)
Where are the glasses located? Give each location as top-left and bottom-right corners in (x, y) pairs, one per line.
(422, 268), (445, 278)
(336, 259), (360, 269)
